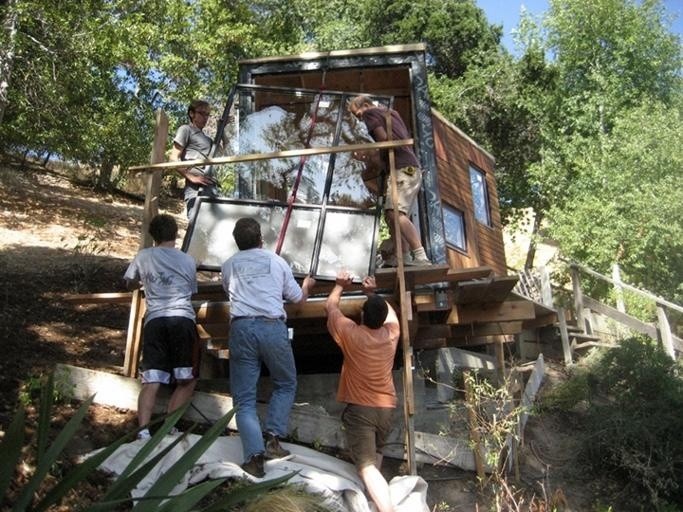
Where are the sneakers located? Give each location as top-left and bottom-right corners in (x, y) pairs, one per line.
(381, 254), (412, 268)
(137, 429), (154, 441)
(167, 426), (179, 434)
(399, 256), (433, 267)
(240, 453), (268, 479)
(261, 437), (294, 465)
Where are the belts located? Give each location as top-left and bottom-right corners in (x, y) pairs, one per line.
(230, 314), (284, 323)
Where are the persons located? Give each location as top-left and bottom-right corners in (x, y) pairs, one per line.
(323, 273), (402, 512)
(220, 216), (316, 477)
(349, 94), (432, 270)
(168, 100), (235, 220)
(123, 214), (198, 441)
